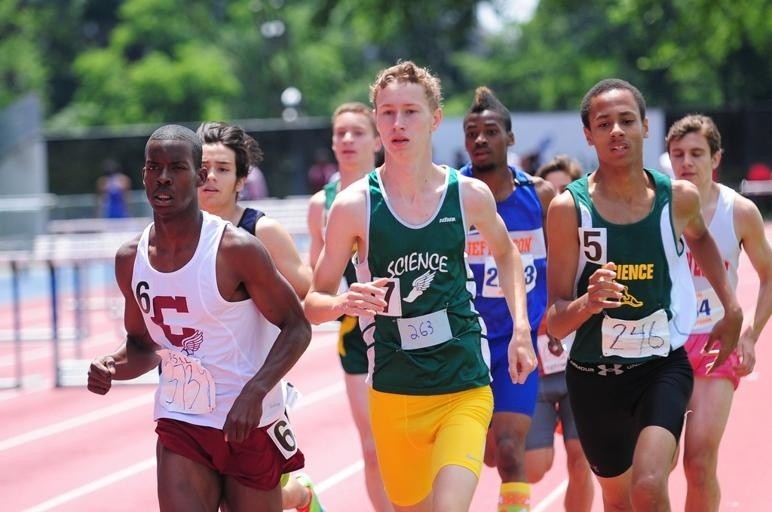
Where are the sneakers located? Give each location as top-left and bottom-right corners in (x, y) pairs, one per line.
(293, 474), (322, 512)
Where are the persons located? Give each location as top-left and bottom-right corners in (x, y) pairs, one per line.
(532, 76), (772, 512)
(87, 121), (325, 512)
(305, 61), (555, 511)
(87, 158), (771, 218)
